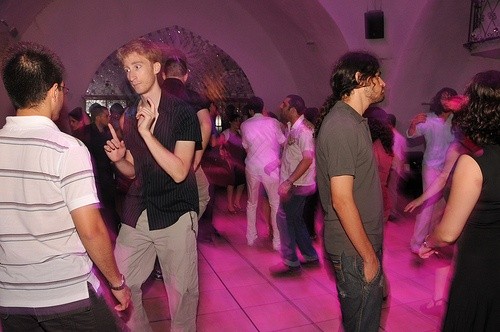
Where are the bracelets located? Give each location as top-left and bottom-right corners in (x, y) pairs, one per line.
(423, 234), (428, 246)
(286, 180), (293, 185)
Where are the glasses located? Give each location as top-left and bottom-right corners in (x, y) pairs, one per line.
(59, 86), (69, 95)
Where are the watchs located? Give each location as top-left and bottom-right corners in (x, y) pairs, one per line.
(111, 273), (126, 291)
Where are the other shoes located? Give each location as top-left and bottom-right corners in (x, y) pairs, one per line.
(298, 258), (320, 267)
(269, 261), (301, 275)
(154, 270), (163, 281)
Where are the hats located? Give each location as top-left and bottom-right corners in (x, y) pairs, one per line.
(67, 107), (82, 122)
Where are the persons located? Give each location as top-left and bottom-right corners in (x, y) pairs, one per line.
(262, 95), (320, 279)
(103, 37), (203, 332)
(205, 99), (318, 147)
(406, 70), (500, 332)
(362, 107), (407, 223)
(219, 115), (246, 214)
(0, 41), (133, 332)
(240, 97), (287, 250)
(313, 51), (386, 332)
(67, 102), (124, 139)
(153, 57), (212, 281)
(72, 106), (116, 230)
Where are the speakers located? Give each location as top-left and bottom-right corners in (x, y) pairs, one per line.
(365, 11), (384, 39)
